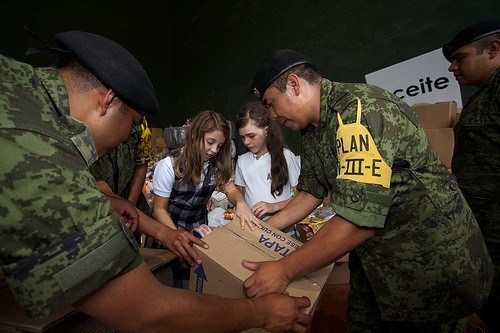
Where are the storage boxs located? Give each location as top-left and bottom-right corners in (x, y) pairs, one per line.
(188, 216), (335, 333)
(148, 119), (236, 172)
(297, 217), (350, 263)
(317, 261), (351, 327)
(407, 101), (461, 172)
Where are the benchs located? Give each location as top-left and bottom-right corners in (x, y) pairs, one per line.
(1, 248), (177, 333)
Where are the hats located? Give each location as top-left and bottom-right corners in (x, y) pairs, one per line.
(55, 30), (160, 116)
(442, 21), (500, 59)
(247, 49), (311, 102)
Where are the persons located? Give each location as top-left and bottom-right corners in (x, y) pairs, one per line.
(88, 115), (209, 267)
(233, 100), (301, 241)
(144, 111), (262, 288)
(0, 32), (312, 333)
(442, 20), (500, 333)
(242, 49), (494, 333)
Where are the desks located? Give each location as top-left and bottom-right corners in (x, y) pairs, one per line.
(31, 249), (187, 333)
